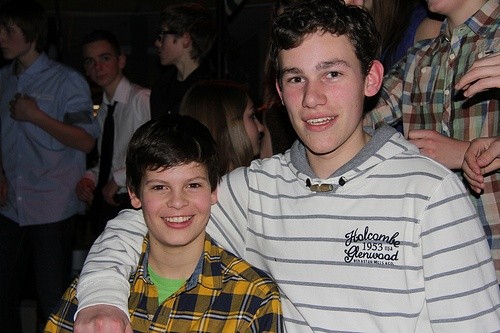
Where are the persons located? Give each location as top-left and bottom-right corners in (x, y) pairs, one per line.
(45, 112), (282, 333)
(0, 0), (500, 333)
(75, 0), (500, 333)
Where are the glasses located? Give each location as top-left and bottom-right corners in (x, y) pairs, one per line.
(159, 30), (175, 40)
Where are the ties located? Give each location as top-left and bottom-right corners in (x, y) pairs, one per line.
(94, 102), (119, 193)
